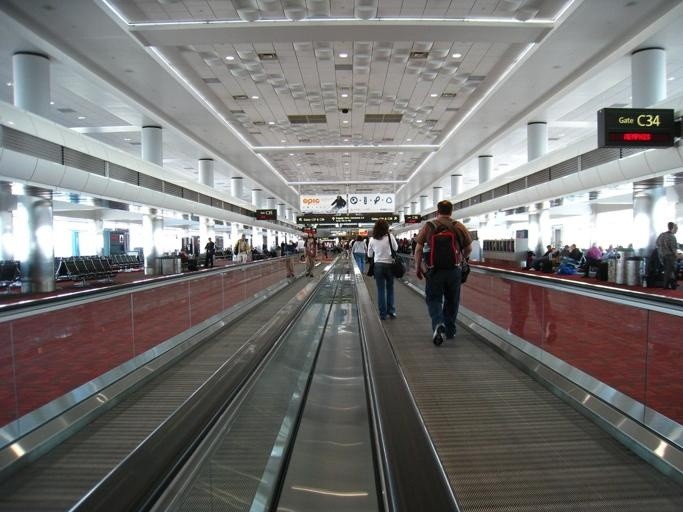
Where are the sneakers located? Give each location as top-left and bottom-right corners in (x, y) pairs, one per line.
(432, 323), (445, 346)
(389, 312), (396, 319)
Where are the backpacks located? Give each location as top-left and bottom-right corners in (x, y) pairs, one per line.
(427, 221), (459, 271)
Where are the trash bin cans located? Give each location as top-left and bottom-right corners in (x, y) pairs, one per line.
(607, 248), (644, 286)
(154, 256), (181, 275)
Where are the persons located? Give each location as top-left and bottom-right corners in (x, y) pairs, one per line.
(204, 238), (215, 267)
(414, 201), (473, 347)
(523, 243), (634, 282)
(235, 234), (249, 263)
(501, 278), (556, 345)
(317, 233), (416, 274)
(655, 222), (679, 291)
(281, 241), (284, 256)
(303, 232), (316, 277)
(285, 258), (295, 278)
(329, 195), (347, 212)
(366, 219), (399, 322)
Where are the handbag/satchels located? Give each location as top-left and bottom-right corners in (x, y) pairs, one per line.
(394, 255), (406, 278)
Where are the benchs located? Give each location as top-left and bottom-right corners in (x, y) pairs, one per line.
(0, 253), (147, 295)
